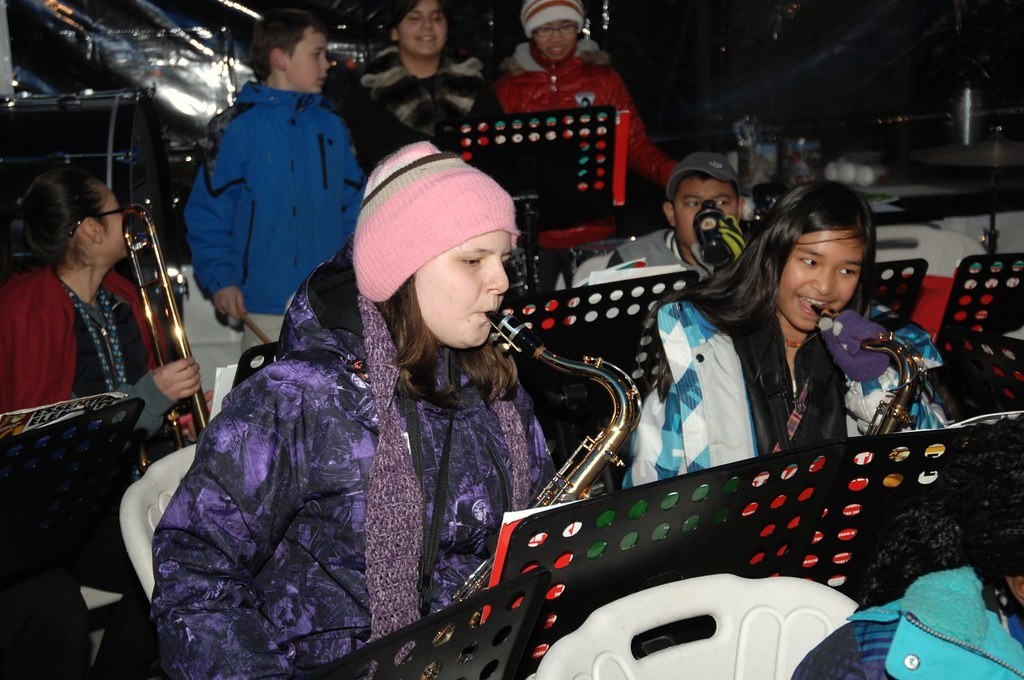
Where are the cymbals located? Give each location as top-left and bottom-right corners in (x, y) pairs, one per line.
(908, 136), (1024, 171)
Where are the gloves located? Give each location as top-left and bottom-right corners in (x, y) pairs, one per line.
(690, 212), (746, 275)
(818, 309), (890, 382)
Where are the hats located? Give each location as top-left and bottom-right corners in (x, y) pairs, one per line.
(520, 0), (585, 38)
(353, 140), (521, 303)
(666, 152), (740, 201)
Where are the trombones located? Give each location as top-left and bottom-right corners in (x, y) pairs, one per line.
(121, 205), (208, 450)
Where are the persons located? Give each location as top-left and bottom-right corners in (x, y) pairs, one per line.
(149, 139), (557, 680)
(1, 163), (205, 680)
(570, 152), (745, 289)
(184, 9), (367, 351)
(623, 181), (949, 494)
(356, 0), (679, 293)
(790, 418), (1023, 680)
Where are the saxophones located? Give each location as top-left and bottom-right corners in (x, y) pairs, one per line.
(422, 311), (643, 678)
(817, 305), (926, 434)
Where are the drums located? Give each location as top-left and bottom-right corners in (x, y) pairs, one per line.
(0, 82), (176, 286)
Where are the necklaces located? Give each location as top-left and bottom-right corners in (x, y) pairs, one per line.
(785, 338), (803, 348)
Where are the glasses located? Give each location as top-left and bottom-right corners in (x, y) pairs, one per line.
(534, 24), (578, 37)
(69, 203), (126, 238)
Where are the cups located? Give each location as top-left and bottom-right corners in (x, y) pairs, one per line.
(693, 199), (731, 267)
